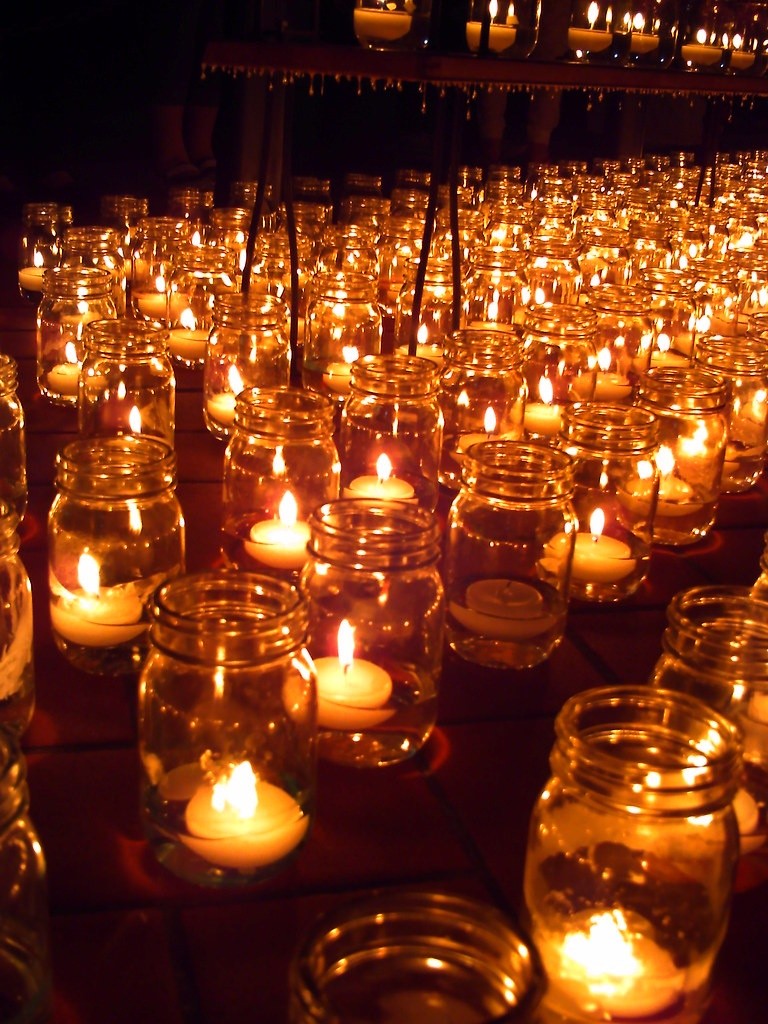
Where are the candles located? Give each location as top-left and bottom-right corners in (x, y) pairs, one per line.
(16, 152), (768, 1017)
(353, 0), (768, 70)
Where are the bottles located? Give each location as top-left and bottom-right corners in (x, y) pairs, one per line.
(0, 0), (768, 1024)
